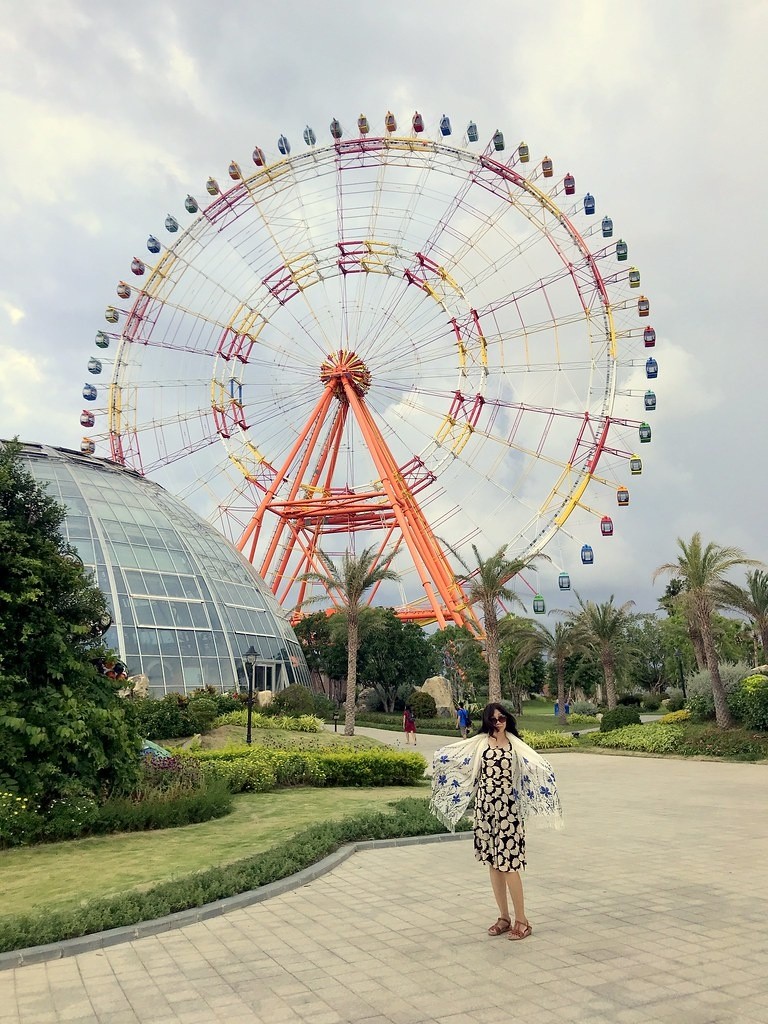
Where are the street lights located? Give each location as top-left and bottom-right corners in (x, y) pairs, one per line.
(674, 649), (687, 704)
(242, 646), (261, 746)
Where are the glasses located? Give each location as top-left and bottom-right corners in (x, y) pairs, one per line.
(491, 715), (506, 725)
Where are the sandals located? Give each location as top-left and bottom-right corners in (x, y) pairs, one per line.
(488, 918), (512, 936)
(508, 921), (532, 940)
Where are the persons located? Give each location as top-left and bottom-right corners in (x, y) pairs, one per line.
(403, 704), (416, 746)
(456, 702), (468, 740)
(428, 702), (562, 940)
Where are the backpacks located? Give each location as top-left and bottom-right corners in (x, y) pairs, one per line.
(461, 709), (472, 728)
(405, 710), (416, 722)
